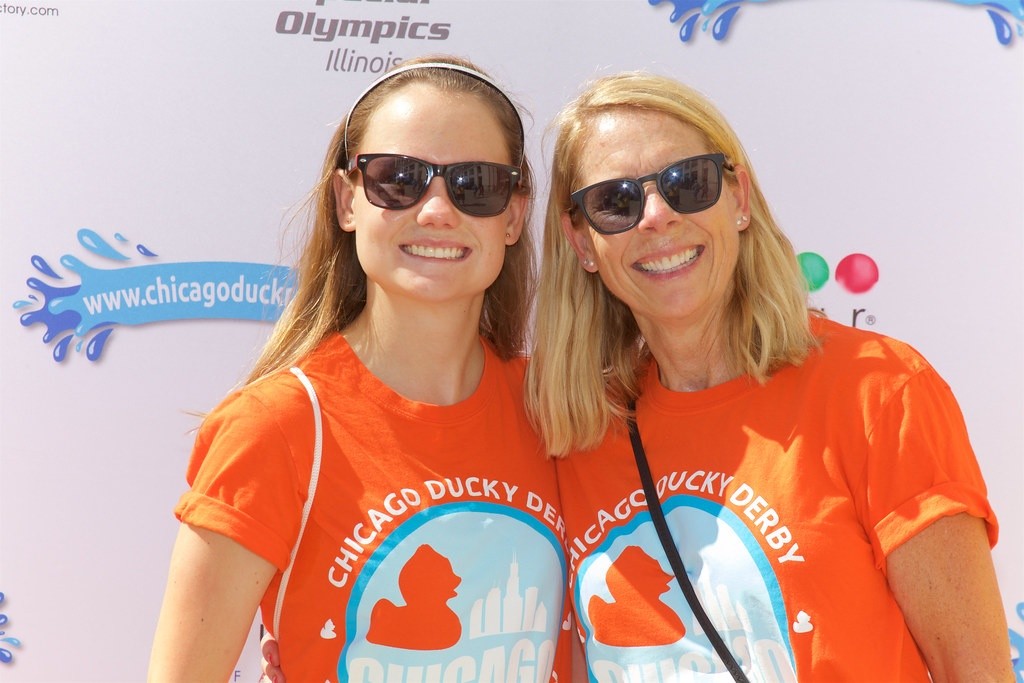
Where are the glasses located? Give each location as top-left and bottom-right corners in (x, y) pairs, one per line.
(571, 153), (734, 236)
(345, 153), (524, 218)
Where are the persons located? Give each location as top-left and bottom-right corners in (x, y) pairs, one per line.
(262, 74), (1017, 682)
(146, 57), (589, 683)
